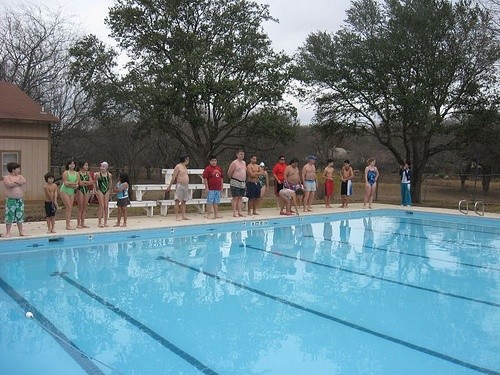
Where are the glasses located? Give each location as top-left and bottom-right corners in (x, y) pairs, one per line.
(280, 159), (285, 161)
(261, 166), (264, 167)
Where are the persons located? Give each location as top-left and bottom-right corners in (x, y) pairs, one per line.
(363, 158), (379, 209)
(44, 173), (57, 233)
(284, 157), (301, 213)
(114, 173), (130, 227)
(247, 155), (263, 215)
(167, 154), (191, 220)
(322, 159), (335, 208)
(302, 156), (317, 212)
(340, 159), (354, 208)
(4, 162), (26, 238)
(257, 162), (269, 211)
(77, 160), (93, 228)
(399, 163), (412, 208)
(203, 155), (224, 219)
(279, 188), (303, 217)
(273, 156), (288, 210)
(60, 159), (80, 230)
(227, 150), (246, 217)
(95, 162), (112, 227)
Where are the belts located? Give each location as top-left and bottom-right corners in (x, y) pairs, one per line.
(231, 177), (244, 182)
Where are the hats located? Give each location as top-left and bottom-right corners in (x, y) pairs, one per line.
(260, 161), (265, 166)
(305, 155), (317, 160)
